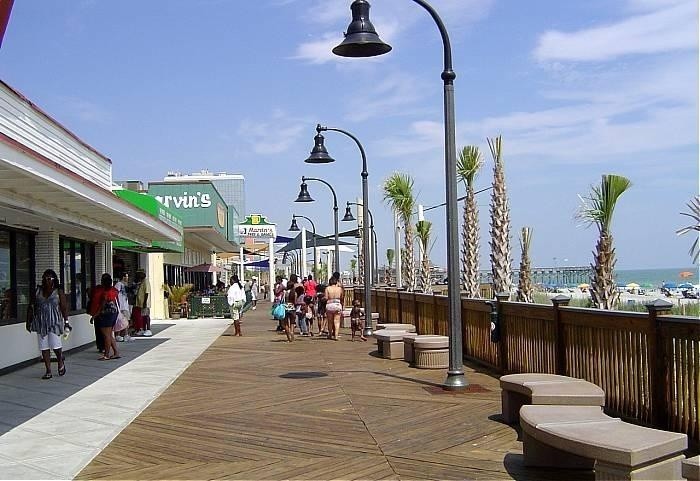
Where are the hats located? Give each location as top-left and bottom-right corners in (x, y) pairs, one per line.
(137, 269), (146, 274)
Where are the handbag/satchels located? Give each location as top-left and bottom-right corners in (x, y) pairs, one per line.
(274, 303), (285, 320)
(114, 311), (129, 332)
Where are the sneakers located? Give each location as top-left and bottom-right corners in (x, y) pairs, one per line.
(124, 335), (135, 341)
(136, 328), (145, 335)
(143, 329), (152, 335)
(116, 335), (124, 341)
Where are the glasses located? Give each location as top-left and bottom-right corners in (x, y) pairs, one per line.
(42, 276), (54, 280)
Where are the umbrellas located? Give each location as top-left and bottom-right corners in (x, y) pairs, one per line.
(576, 283), (590, 294)
(615, 282), (695, 296)
(185, 262), (228, 289)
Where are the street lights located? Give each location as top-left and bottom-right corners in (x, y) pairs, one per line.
(332, 0), (471, 391)
(304, 124), (374, 337)
(282, 242), (302, 277)
(289, 213), (317, 283)
(341, 200), (374, 287)
(294, 175), (340, 286)
(355, 223), (378, 284)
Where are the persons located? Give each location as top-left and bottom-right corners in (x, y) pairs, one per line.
(271, 272), (368, 343)
(86, 269), (152, 362)
(193, 277), (271, 311)
(27, 269), (70, 379)
(226, 276), (247, 337)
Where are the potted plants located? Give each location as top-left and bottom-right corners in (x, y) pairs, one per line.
(160, 281), (194, 319)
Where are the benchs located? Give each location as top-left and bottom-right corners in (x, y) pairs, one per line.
(516, 402), (690, 481)
(372, 318), (417, 361)
(341, 305), (381, 330)
(402, 328), (454, 370)
(677, 451), (700, 481)
(497, 368), (604, 430)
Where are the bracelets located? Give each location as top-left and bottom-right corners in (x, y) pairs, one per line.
(64, 320), (69, 325)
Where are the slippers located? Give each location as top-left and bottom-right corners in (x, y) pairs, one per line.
(42, 373), (52, 379)
(110, 355), (122, 358)
(97, 356), (110, 359)
(58, 360), (65, 375)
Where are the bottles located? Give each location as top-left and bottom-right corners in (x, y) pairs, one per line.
(63, 328), (70, 341)
